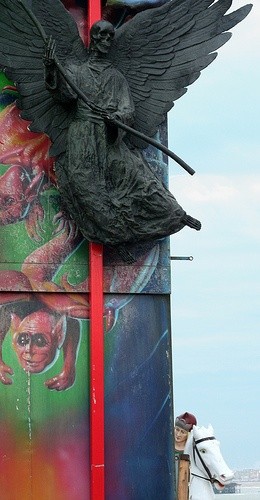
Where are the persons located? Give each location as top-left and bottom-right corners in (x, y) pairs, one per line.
(174, 411), (197, 452)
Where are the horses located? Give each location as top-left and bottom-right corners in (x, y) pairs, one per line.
(184, 424), (235, 500)
